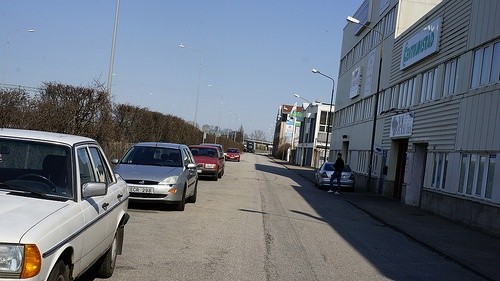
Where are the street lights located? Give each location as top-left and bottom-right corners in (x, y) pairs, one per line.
(311, 69), (335, 164)
(294, 93), (312, 166)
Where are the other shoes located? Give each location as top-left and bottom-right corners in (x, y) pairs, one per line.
(328, 190), (334, 192)
(334, 192), (340, 194)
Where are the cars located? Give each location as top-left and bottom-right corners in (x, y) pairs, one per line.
(110, 144), (204, 211)
(187, 145), (225, 181)
(225, 148), (241, 162)
(0, 128), (130, 281)
(315, 161), (356, 189)
(200, 143), (226, 163)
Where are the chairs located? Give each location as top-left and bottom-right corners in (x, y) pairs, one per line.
(191, 150), (198, 156)
(160, 153), (179, 162)
(42, 155), (69, 186)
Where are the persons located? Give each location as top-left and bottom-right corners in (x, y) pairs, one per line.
(327, 153), (344, 195)
